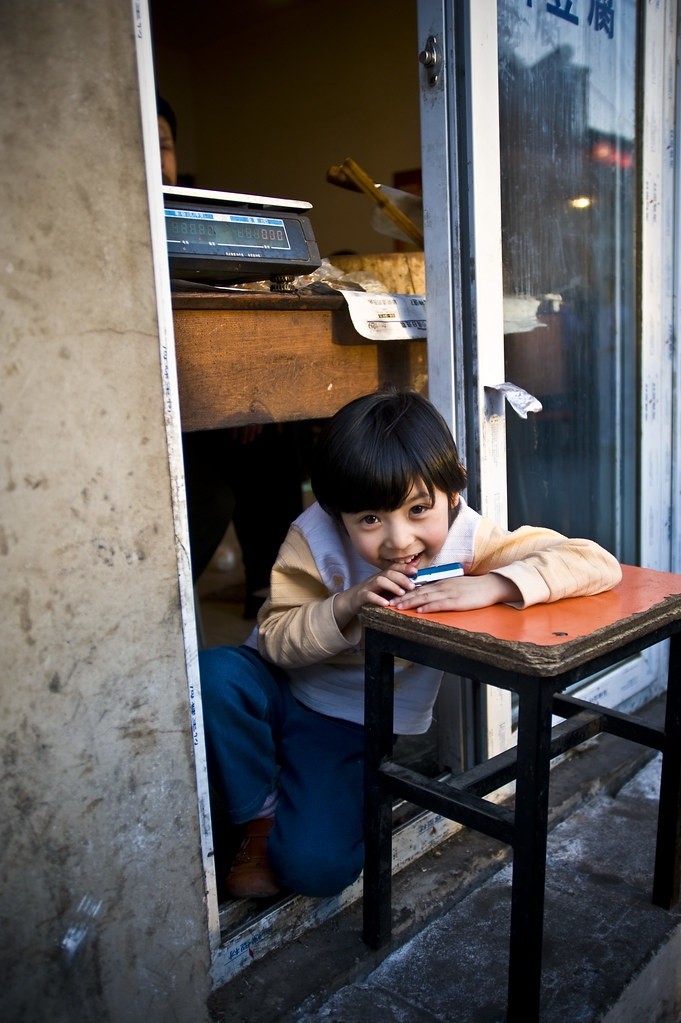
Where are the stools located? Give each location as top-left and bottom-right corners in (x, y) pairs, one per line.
(358, 563), (681, 1023)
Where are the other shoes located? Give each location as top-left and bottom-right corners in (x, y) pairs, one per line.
(228, 819), (279, 897)
(241, 592), (268, 620)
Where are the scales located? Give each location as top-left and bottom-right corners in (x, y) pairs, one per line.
(164, 184), (322, 283)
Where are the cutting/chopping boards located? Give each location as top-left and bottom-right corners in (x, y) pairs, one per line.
(317, 252), (424, 297)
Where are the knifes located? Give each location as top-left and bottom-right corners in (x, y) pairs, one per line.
(325, 165), (424, 250)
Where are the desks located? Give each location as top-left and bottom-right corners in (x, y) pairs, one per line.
(173, 307), (577, 527)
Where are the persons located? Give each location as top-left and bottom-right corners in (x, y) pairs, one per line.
(157, 92), (305, 620)
(198, 384), (623, 898)
